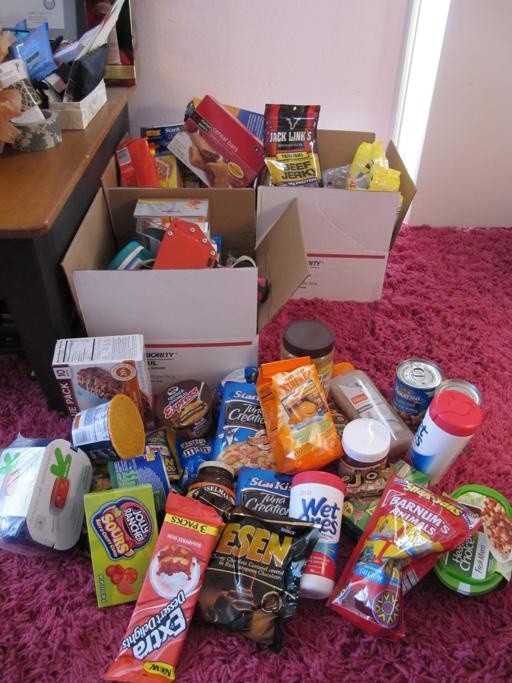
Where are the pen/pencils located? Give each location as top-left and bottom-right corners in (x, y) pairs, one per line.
(52, 35), (65, 53)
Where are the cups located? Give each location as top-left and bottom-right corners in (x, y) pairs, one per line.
(64, 391), (143, 464)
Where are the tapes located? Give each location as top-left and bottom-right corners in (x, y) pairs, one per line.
(9, 108), (61, 153)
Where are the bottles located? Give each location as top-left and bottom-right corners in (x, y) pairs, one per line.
(326, 361), (414, 459)
(337, 419), (393, 478)
(187, 460), (239, 515)
(407, 390), (485, 488)
(282, 470), (348, 602)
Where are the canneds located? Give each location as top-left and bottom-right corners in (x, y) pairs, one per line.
(186, 460), (237, 509)
(340, 420), (391, 486)
(393, 360), (484, 434)
(282, 320), (335, 407)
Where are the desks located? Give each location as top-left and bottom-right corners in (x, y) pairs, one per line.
(0, 94), (129, 418)
